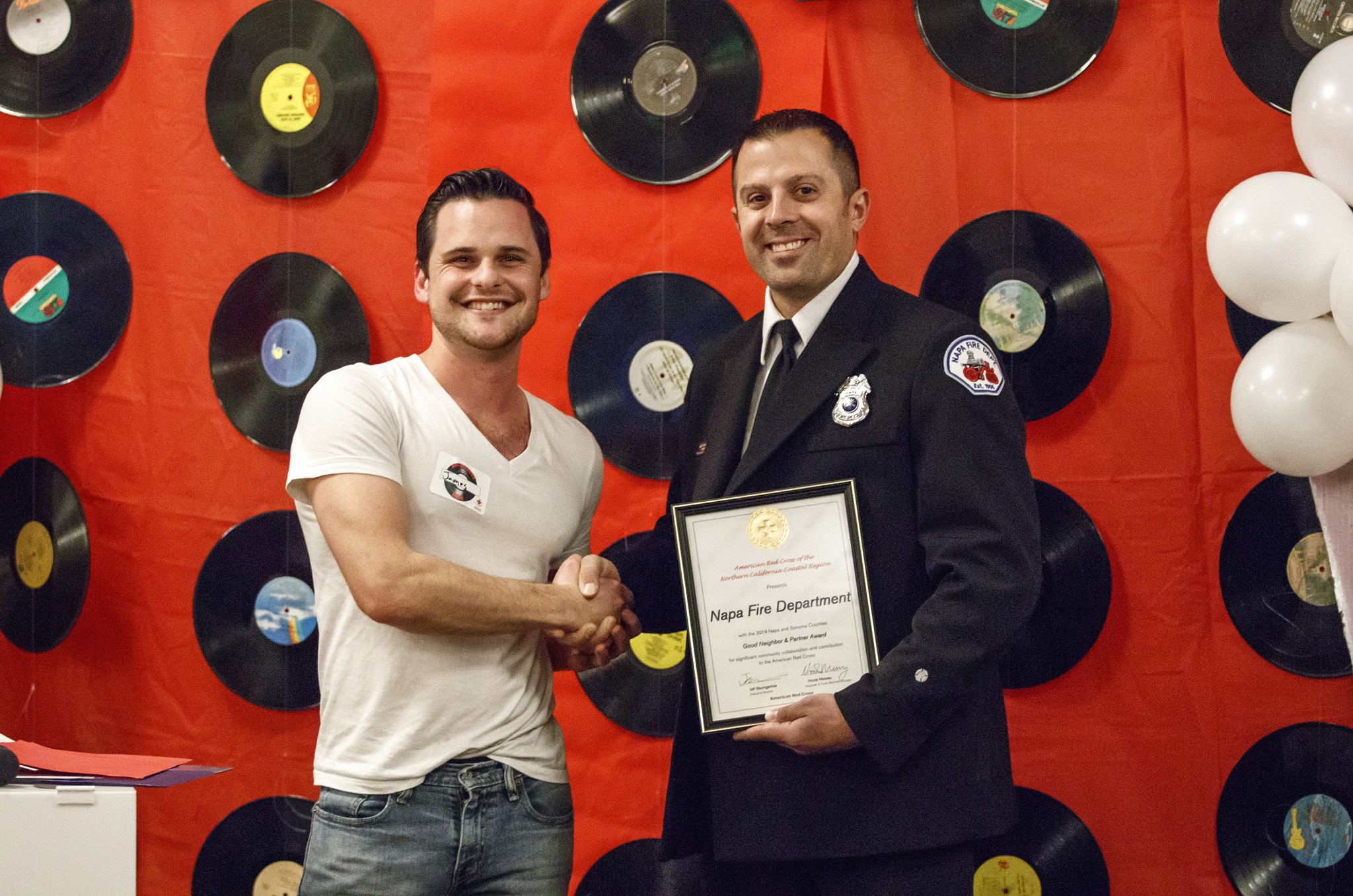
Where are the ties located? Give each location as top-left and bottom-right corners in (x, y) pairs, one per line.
(748, 319), (801, 449)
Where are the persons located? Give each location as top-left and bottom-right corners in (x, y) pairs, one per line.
(540, 106), (1045, 896)
(284, 164), (636, 896)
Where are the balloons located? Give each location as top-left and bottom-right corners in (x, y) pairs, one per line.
(1205, 32), (1353, 480)
